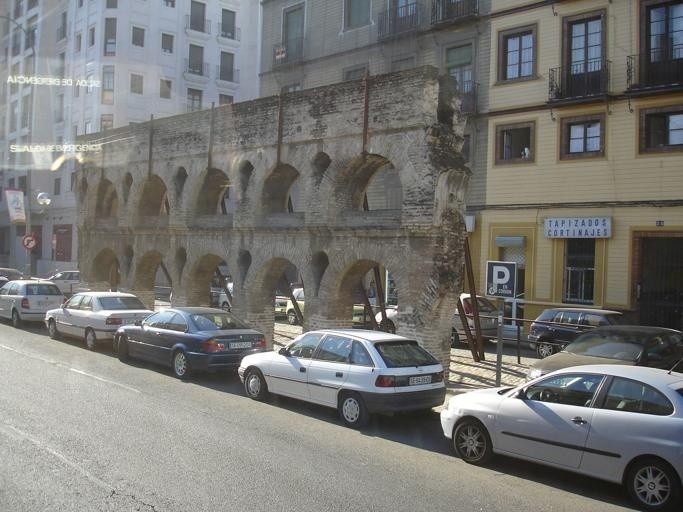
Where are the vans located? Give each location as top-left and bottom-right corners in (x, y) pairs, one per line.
(527, 308), (623, 358)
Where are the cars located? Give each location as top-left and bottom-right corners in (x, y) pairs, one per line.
(44, 291), (153, 350)
(0, 268), (80, 328)
(238, 328), (446, 427)
(526, 325), (683, 384)
(154, 283), (287, 316)
(374, 293), (498, 348)
(285, 287), (371, 329)
(114, 307), (266, 380)
(440, 360), (683, 512)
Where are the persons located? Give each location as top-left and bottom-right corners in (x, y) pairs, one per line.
(366, 280), (378, 316)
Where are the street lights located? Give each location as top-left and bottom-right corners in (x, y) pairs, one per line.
(23, 192), (52, 280)
